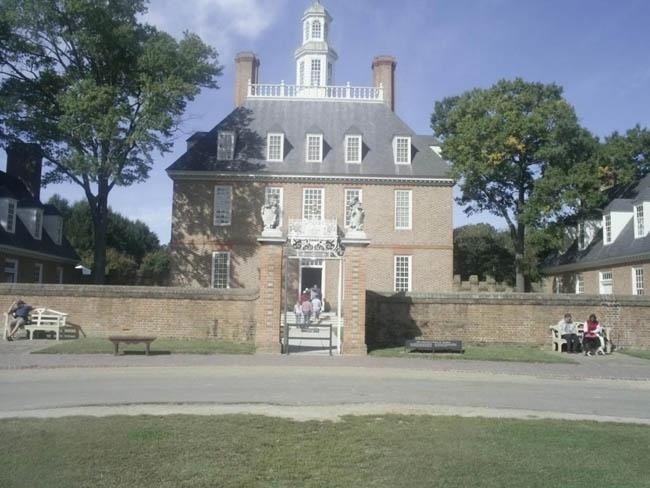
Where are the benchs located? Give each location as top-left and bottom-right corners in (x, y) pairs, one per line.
(108, 334), (157, 356)
(0, 306), (70, 343)
(550, 322), (613, 354)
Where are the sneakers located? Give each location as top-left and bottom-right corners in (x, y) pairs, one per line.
(4, 335), (14, 343)
(566, 349), (579, 355)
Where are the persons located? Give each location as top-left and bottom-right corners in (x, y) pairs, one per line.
(4, 300), (36, 340)
(556, 313), (580, 353)
(293, 284), (322, 329)
(582, 314), (603, 356)
(322, 299), (331, 314)
